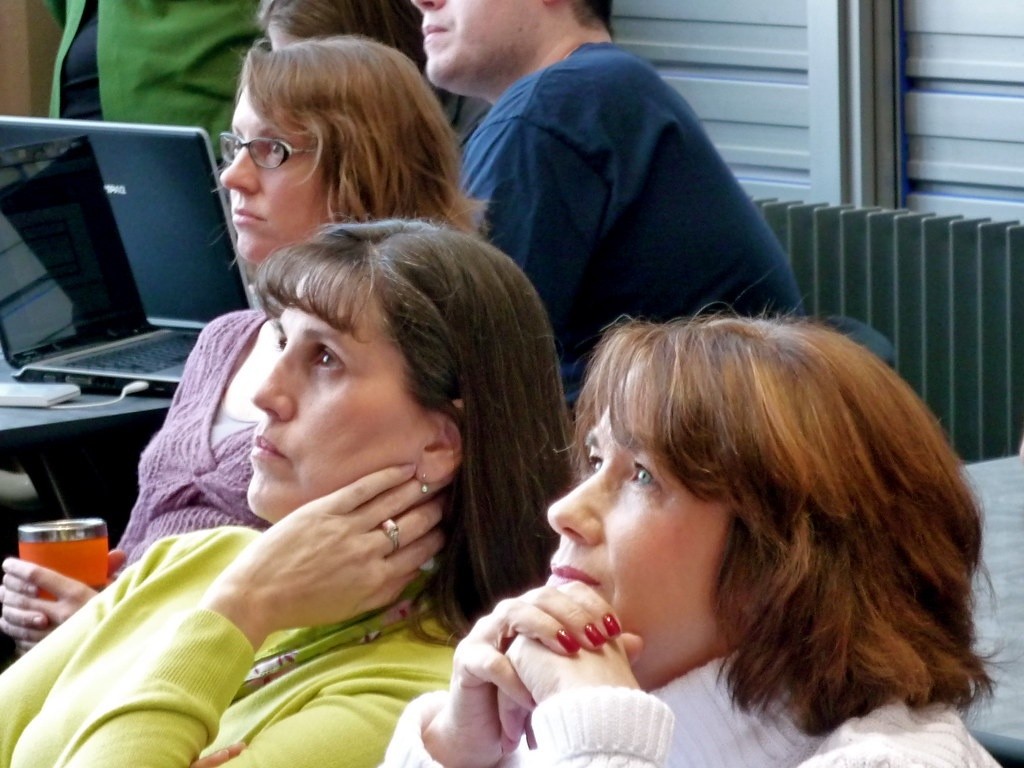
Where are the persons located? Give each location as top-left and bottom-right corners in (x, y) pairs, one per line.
(376, 301), (1004, 768)
(0, 218), (571, 768)
(413, 0), (809, 416)
(1, 36), (460, 659)
(257, 0), (490, 161)
(39, 0), (266, 163)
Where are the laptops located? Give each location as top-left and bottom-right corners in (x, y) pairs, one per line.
(0, 116), (255, 401)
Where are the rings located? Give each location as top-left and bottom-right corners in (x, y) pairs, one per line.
(381, 518), (400, 552)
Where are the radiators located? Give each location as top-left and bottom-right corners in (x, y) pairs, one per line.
(754, 199), (1024, 461)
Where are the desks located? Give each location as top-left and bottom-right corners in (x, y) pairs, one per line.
(0, 360), (176, 552)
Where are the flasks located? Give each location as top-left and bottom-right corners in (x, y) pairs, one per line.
(18, 516), (109, 603)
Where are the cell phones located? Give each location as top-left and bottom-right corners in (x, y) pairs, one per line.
(0, 383), (81, 407)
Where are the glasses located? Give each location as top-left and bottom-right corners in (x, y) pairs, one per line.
(217, 130), (313, 169)
